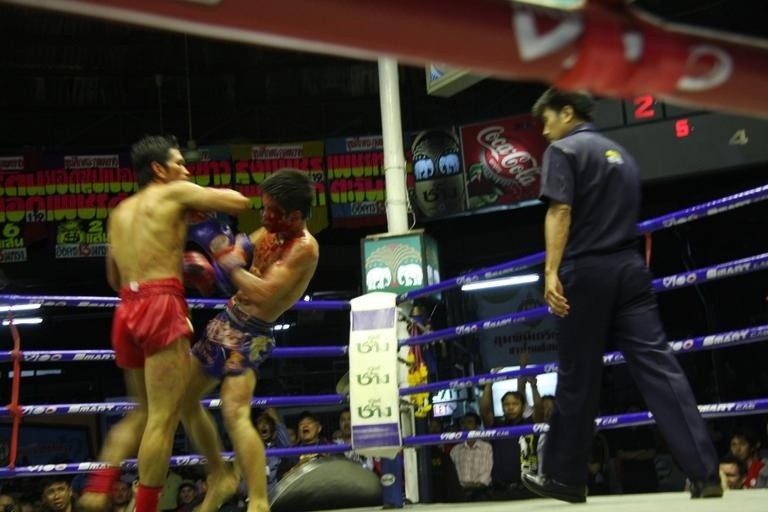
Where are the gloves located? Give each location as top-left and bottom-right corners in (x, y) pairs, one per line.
(183, 220), (253, 296)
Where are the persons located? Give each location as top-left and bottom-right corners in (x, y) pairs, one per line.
(522, 81), (724, 506)
(721, 426), (768, 490)
(74, 134), (265, 510)
(237, 406), (353, 510)
(428, 367), (659, 502)
(0, 474), (208, 512)
(180, 169), (321, 508)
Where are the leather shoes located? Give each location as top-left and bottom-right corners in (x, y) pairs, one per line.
(690, 476), (722, 498)
(522, 473), (588, 502)
